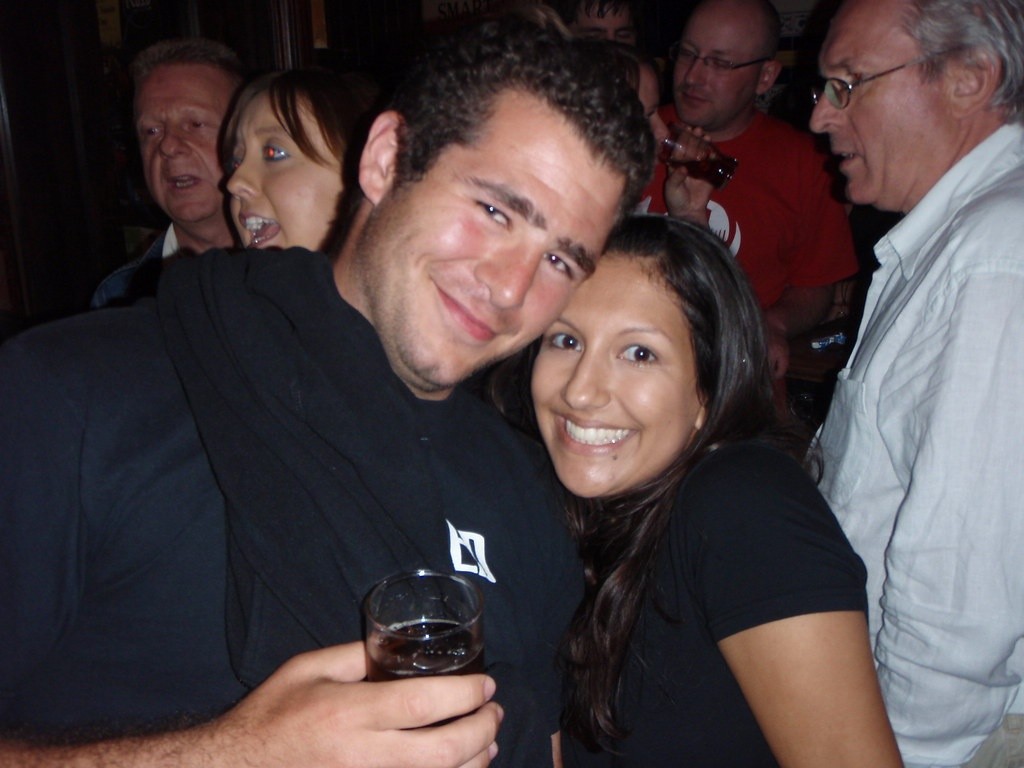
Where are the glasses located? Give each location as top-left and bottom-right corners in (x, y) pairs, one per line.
(676, 47), (771, 76)
(810, 47), (965, 110)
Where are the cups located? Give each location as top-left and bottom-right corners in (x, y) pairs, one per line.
(657, 122), (739, 191)
(361, 568), (486, 730)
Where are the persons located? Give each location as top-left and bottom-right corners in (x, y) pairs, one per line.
(0, 0), (1024, 768)
